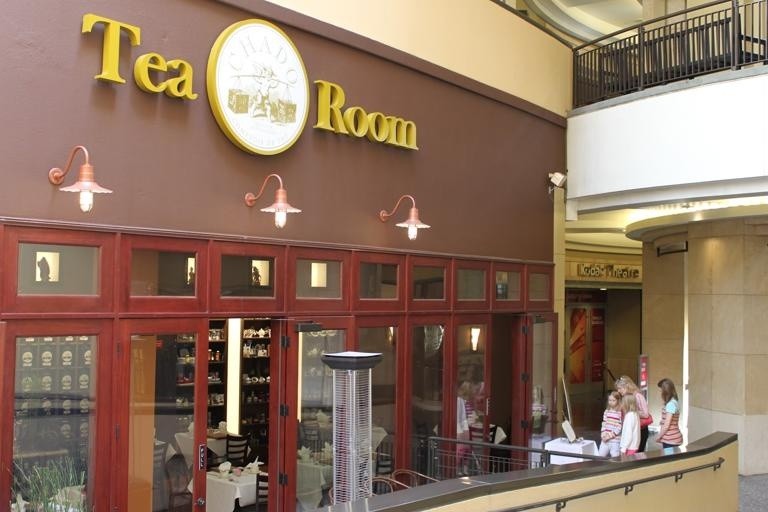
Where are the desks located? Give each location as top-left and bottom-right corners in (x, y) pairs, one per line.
(435, 424), (508, 446)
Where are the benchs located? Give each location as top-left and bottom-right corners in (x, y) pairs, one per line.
(598, 11), (768, 101)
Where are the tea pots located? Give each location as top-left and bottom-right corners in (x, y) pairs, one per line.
(255, 328), (268, 337)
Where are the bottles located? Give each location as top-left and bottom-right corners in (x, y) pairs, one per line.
(209, 329), (224, 341)
(208, 349), (224, 362)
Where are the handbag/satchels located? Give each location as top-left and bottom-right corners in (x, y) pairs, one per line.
(640, 414), (652, 429)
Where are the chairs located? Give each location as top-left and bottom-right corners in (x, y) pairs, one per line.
(373, 441), (394, 494)
(162, 455), (194, 511)
(414, 419), (427, 447)
(468, 425), (496, 447)
(243, 475), (268, 511)
(225, 432), (251, 467)
(301, 423), (321, 450)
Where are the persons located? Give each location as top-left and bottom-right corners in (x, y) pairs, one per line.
(615, 374), (652, 453)
(456, 381), (484, 466)
(597, 390), (621, 459)
(619, 393), (642, 456)
(531, 385), (546, 415)
(656, 377), (684, 447)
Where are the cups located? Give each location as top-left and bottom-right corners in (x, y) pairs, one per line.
(245, 375), (270, 384)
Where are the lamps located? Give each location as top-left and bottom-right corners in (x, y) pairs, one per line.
(470, 328), (480, 352)
(244, 173), (301, 228)
(47, 144), (116, 213)
(379, 194), (430, 241)
